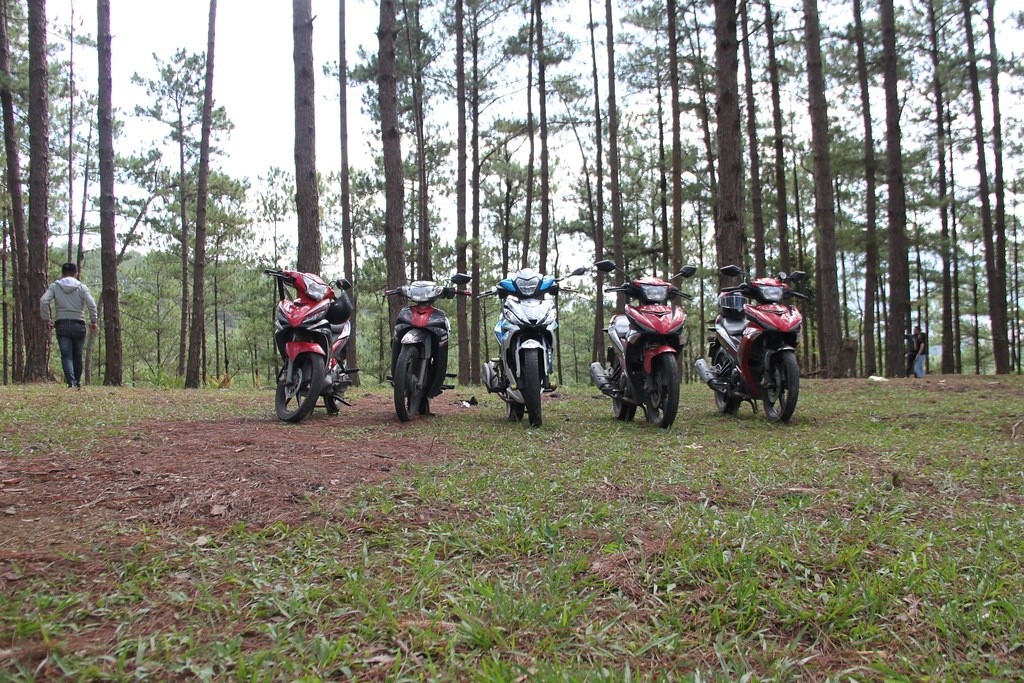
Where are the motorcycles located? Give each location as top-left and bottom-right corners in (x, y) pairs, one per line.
(694, 265), (810, 428)
(587, 259), (697, 429)
(263, 269), (362, 423)
(385, 272), (473, 422)
(476, 266), (590, 429)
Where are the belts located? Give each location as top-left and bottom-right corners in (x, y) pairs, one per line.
(55, 319), (86, 325)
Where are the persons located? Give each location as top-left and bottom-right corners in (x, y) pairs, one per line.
(40, 263), (98, 390)
(905, 326), (926, 378)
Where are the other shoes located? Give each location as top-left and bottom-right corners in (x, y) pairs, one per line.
(67, 380), (81, 388)
